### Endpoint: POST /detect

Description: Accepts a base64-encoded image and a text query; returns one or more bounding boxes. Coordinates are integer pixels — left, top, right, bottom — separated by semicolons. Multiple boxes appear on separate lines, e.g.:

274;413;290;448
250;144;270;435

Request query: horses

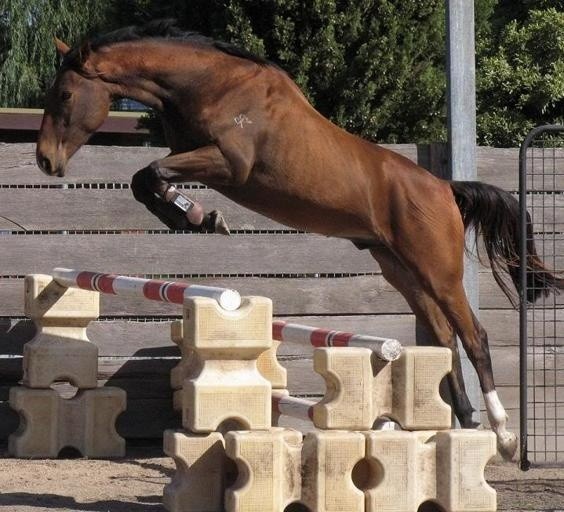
36;11;564;461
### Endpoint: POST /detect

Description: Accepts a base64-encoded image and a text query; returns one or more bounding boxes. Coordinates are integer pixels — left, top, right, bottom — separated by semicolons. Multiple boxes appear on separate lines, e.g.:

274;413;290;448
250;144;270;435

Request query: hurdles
9;267;498;512
